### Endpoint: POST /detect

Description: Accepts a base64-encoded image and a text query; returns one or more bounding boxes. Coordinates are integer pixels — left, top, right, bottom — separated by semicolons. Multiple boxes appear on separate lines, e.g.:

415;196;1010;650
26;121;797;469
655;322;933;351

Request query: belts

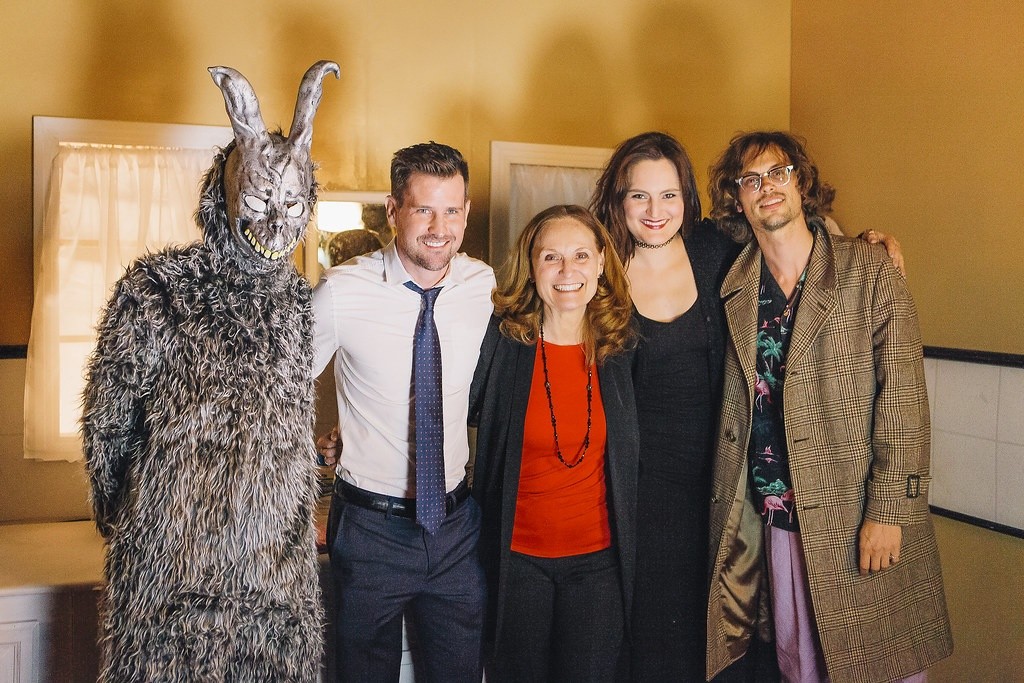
331;476;472;520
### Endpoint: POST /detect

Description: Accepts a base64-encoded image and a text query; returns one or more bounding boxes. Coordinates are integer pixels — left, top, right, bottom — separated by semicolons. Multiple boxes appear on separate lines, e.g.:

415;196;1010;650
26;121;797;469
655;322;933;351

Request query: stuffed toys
81;59;320;683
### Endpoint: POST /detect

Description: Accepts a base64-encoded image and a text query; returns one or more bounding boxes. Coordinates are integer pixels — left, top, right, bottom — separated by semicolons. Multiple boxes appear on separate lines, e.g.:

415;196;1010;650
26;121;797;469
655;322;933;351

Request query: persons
312;131;932;683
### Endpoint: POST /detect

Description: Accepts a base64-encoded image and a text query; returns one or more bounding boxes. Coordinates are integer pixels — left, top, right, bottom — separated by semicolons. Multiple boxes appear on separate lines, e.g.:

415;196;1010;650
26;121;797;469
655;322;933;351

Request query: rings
889;556;900;559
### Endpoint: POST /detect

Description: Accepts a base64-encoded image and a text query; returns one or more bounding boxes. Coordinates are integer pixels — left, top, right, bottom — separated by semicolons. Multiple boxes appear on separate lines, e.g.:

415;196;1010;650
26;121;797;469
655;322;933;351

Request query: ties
402;280;446;536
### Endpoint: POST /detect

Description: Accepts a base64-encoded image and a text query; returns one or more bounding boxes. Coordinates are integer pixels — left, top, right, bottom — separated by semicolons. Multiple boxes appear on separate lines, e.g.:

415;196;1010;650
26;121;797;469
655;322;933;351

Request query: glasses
733;166;795;195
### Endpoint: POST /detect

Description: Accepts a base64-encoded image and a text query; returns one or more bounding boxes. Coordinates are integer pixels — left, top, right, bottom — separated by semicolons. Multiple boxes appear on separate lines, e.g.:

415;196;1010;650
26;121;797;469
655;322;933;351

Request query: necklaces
540;301;622;468
629;231;679;250
